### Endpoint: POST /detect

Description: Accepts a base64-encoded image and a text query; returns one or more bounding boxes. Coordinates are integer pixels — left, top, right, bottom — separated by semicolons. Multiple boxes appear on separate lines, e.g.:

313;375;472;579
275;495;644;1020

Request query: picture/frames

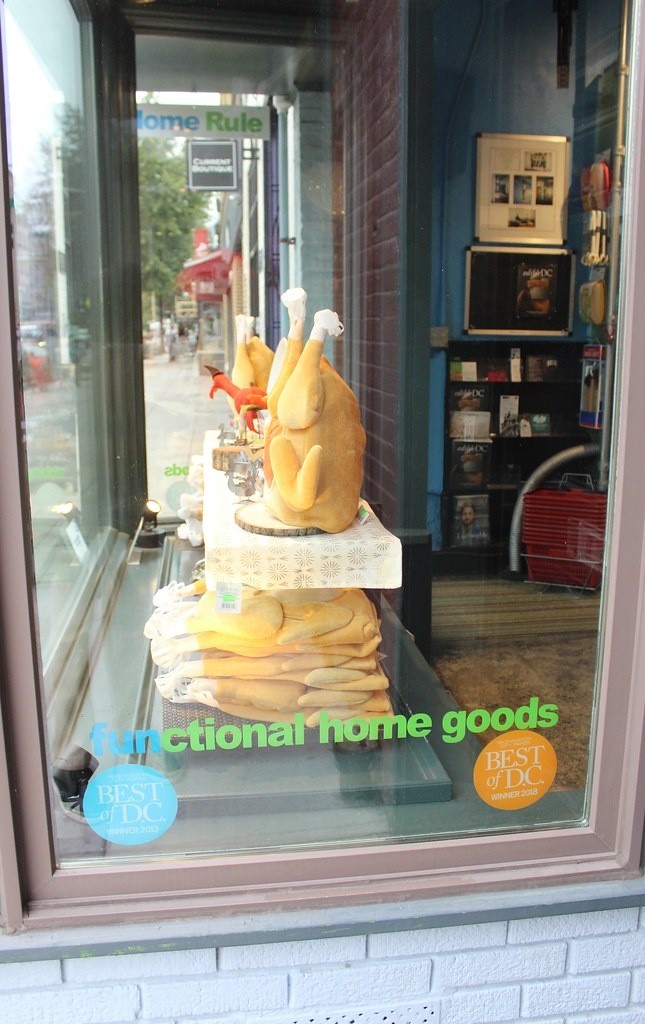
463;244;577;337
473;131;571;245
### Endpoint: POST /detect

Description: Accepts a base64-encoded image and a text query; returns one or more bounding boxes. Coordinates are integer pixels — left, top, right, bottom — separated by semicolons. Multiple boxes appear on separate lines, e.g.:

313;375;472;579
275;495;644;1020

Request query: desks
201;428;403;754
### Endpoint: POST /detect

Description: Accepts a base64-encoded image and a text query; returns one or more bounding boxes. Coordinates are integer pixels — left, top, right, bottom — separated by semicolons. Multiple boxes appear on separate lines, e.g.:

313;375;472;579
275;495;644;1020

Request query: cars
143;334;159;357
19;321;92;381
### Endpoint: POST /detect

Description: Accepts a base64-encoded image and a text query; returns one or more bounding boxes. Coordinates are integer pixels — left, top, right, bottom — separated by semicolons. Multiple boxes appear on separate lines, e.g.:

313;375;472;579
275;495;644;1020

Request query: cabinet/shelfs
439;341;588;548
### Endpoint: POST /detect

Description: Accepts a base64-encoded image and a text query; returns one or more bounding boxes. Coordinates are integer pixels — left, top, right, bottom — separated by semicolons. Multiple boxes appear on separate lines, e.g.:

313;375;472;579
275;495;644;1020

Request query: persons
42;630;125;824
452;502;489;546
164;312;176;360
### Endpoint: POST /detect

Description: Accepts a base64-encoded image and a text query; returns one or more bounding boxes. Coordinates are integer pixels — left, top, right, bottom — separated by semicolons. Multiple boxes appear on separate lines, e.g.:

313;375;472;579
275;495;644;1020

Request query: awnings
177;251;236;303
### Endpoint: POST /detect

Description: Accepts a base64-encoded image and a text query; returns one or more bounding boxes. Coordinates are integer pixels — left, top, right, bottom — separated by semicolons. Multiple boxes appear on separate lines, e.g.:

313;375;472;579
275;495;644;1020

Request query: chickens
147;564;392;736
211;285;367;535
